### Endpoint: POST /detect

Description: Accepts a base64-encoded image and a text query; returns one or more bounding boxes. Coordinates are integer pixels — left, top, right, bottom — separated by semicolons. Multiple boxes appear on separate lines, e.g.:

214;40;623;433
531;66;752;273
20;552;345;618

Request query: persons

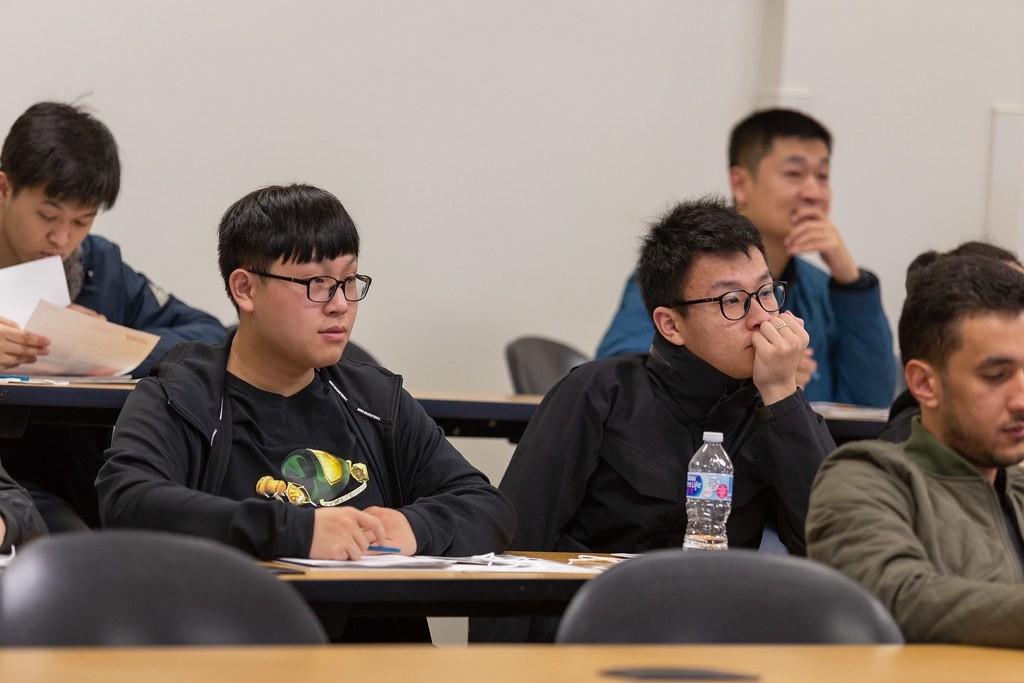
0;102;230;565
467;196;838;645
805;241;1024;649
596;108;898;409
95;182;517;646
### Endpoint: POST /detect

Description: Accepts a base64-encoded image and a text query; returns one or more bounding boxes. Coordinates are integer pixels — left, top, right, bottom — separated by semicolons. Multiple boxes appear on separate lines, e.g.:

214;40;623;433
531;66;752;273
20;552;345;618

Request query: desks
0;645;1024;683
251;549;754;618
0;375;890;444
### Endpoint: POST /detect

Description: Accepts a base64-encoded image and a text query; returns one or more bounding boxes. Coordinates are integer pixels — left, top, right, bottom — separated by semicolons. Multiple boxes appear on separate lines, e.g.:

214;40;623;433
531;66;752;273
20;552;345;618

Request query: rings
777;323;789;329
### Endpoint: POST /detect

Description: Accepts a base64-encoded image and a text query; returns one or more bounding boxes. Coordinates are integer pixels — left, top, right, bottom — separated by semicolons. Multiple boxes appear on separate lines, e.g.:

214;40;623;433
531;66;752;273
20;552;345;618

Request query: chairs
0;530;326;649
505;334;591;396
556;548;903;647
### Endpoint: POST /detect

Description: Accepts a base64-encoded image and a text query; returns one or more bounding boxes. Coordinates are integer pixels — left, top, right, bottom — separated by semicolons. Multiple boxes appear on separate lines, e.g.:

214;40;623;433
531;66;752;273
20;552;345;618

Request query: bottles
683;433;733;551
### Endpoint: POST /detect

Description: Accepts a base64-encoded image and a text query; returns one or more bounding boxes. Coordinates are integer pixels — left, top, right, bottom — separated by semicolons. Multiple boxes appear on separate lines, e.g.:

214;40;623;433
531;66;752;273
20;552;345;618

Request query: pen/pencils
368;546;400;551
813;372;820;380
0;375;30;381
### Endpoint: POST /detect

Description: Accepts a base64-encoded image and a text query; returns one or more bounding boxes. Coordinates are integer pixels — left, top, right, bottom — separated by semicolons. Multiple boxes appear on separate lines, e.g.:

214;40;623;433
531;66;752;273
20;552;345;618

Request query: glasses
246;268;373;303
675;281;789;320
